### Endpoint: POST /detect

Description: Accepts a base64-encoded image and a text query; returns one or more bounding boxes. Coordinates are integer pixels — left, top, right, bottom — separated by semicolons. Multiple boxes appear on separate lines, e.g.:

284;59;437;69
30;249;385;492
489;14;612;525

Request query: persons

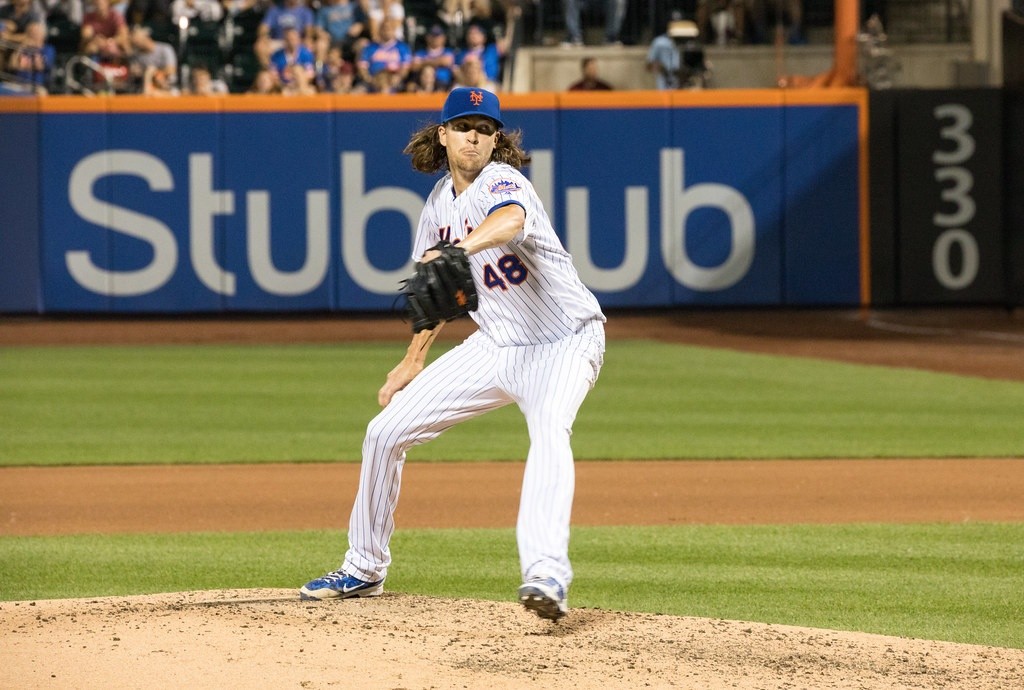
852;13;900;91
566;58;612;91
0;0;546;94
300;86;608;618
645;12;713;89
563;0;808;44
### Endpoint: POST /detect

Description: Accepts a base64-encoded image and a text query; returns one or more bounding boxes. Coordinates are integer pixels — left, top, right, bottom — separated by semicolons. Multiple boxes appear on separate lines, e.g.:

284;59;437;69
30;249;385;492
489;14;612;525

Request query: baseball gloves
392;236;480;333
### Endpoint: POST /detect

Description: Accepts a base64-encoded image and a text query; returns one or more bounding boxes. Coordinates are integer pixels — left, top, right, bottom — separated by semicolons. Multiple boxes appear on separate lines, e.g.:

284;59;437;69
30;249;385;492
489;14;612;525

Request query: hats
441;87;505;128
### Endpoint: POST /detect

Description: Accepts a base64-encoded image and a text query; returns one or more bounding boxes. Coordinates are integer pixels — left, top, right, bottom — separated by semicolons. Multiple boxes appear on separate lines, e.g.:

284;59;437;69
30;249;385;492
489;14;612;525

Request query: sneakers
517;576;568;618
299;568;386;600
